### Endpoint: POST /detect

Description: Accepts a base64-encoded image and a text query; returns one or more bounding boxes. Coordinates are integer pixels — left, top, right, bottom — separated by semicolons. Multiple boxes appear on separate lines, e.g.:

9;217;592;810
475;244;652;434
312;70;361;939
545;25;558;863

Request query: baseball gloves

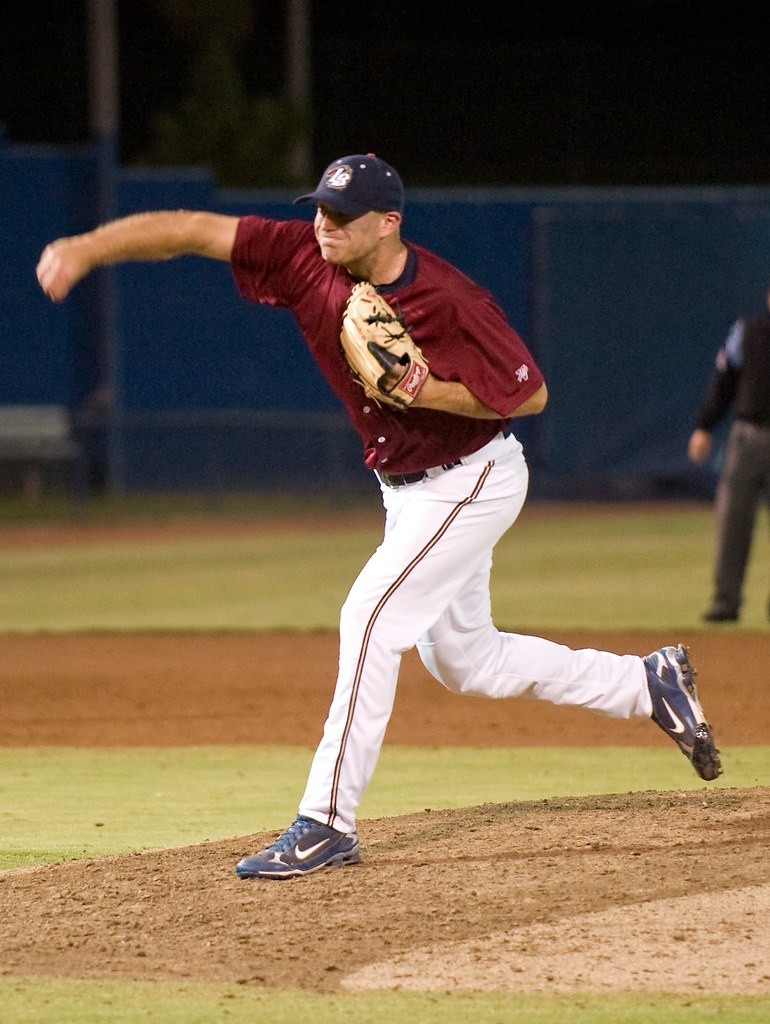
337;282;432;412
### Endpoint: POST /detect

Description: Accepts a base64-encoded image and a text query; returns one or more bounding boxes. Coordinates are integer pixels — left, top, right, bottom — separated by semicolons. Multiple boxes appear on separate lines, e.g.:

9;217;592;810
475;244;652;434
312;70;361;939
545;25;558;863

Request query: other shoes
703;609;737;622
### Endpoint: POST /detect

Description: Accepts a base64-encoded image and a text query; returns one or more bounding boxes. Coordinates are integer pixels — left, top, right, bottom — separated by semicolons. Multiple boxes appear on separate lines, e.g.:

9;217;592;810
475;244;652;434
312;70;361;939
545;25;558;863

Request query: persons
193;0;259;143
35;150;726;881
686;307;769;622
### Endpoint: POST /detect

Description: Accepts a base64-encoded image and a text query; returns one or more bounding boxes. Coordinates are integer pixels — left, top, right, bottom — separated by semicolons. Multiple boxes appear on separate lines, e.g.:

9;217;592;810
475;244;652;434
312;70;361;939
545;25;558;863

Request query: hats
293;153;405;216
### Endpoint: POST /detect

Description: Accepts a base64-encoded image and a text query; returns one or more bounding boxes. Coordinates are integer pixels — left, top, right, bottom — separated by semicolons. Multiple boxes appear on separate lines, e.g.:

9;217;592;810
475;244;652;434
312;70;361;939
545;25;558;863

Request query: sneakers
643;643;723;781
235;817;359;879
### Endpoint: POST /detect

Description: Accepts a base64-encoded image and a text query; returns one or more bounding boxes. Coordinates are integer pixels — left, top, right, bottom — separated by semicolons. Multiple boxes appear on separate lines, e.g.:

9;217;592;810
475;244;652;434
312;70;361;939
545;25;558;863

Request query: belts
376;426;512;489
